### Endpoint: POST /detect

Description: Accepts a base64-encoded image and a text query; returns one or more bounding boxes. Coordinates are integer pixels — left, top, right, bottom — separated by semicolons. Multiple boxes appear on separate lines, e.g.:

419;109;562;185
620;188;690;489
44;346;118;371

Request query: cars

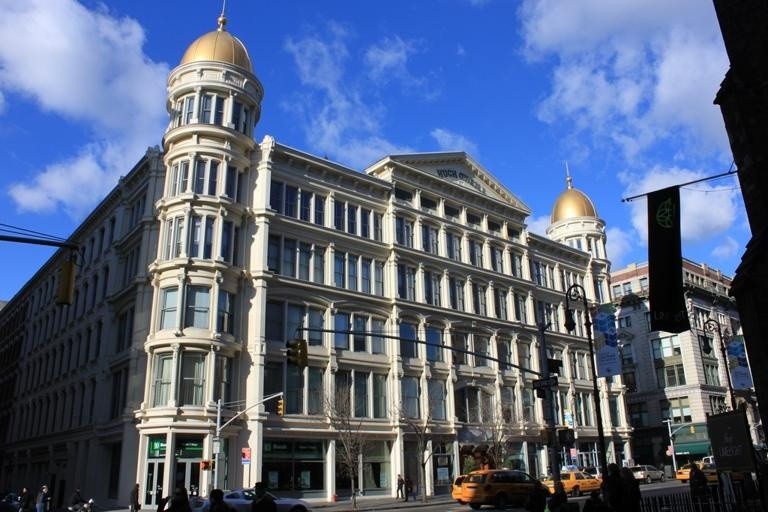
191;489;312;512
676;456;718;483
452;465;666;510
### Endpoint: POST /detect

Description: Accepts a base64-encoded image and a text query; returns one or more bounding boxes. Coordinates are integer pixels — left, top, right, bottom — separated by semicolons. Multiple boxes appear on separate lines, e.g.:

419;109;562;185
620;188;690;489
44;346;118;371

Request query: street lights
564;283;609;481
703;318;736;410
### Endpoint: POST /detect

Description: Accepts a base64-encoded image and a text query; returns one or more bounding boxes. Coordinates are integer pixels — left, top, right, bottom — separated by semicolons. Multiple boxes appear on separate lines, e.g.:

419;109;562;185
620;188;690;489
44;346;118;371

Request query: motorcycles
63;499;95;512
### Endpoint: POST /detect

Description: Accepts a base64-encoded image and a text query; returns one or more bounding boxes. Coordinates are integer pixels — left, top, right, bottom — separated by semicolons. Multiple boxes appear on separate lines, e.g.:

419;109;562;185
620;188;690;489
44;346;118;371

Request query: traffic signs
533;377;558;389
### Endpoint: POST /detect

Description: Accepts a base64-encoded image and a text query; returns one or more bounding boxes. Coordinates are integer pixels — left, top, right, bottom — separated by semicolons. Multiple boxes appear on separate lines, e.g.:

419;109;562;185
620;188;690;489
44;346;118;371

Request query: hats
255;483;268;488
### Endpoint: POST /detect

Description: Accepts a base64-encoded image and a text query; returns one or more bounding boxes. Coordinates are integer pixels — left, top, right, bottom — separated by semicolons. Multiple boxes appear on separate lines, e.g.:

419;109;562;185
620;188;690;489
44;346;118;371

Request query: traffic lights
275;399;286;417
286;339;308;367
691;426;696;434
200;461;211;470
55;263;76;304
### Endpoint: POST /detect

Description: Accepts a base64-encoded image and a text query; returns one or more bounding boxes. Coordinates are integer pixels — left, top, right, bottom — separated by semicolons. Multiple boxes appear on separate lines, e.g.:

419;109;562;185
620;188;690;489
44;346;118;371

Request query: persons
156;486;192;512
17;487;30;512
401;477;417;502
396;474;404;500
547;462;642;512
207;489;230;512
251;481;277;512
689;464;711;512
35;485;52;512
128;484;139;512
71;487;88;512
